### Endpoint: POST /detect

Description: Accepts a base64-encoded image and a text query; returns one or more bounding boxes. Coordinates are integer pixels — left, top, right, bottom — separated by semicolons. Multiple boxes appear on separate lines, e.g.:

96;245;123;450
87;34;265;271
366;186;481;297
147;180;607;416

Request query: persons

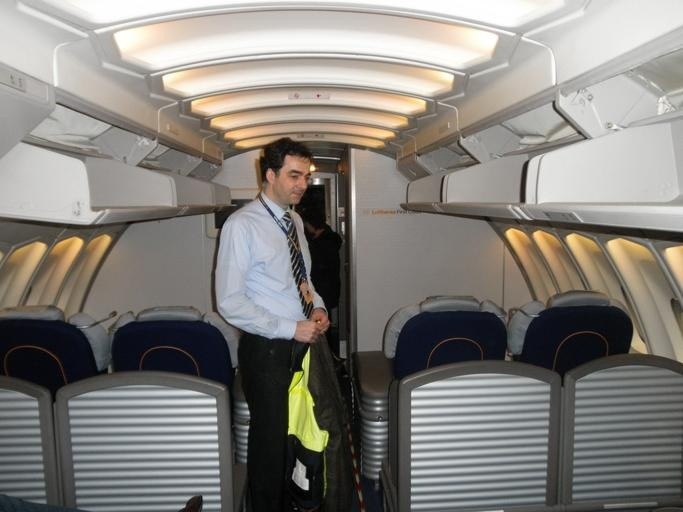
214;137;330;512
304;215;342;349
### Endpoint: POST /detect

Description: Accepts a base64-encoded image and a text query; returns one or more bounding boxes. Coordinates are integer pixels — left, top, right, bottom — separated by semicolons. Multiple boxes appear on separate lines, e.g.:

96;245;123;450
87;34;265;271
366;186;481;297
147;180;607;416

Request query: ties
281;212;314;320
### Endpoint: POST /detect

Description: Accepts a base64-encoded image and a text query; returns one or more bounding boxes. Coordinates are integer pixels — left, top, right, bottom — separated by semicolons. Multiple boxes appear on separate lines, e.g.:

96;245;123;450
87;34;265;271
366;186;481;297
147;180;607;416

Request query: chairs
3;376;63;508
506;289;635;388
380;360;561;511
351;294;508;512
555;354;680;511
4;304;112;405
106;304;251;473
52;371;252;511
